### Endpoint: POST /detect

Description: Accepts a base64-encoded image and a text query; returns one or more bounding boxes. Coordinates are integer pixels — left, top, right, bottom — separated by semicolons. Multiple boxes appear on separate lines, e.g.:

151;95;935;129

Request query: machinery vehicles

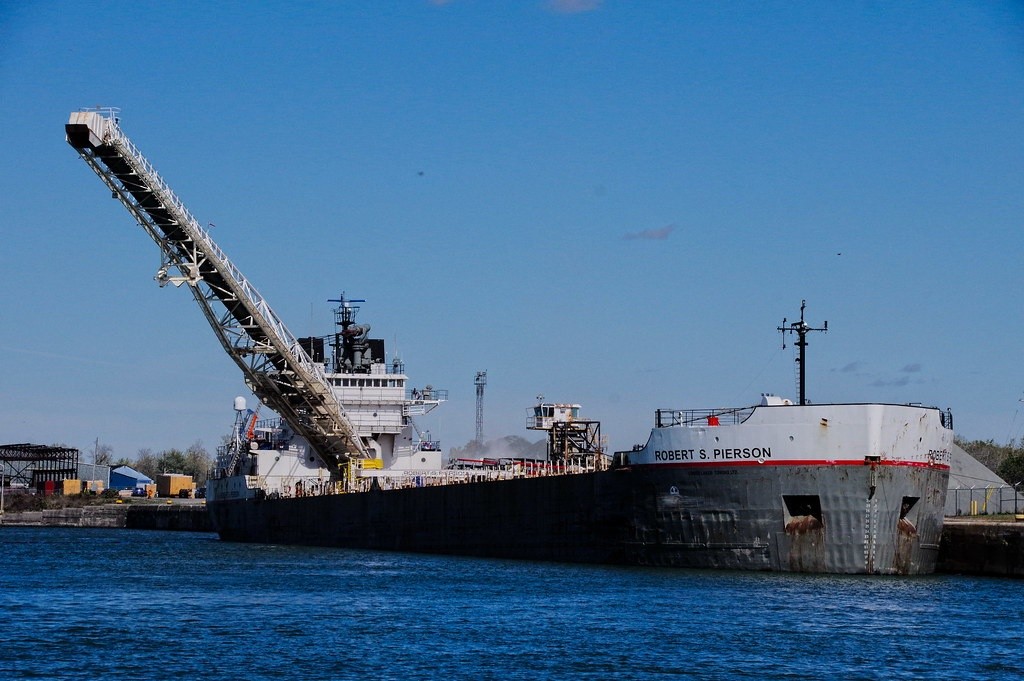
65;105;384;482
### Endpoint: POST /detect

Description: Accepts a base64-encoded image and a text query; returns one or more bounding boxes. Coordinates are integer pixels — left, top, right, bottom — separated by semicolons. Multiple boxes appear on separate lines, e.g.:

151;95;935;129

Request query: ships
204;292;956;578
